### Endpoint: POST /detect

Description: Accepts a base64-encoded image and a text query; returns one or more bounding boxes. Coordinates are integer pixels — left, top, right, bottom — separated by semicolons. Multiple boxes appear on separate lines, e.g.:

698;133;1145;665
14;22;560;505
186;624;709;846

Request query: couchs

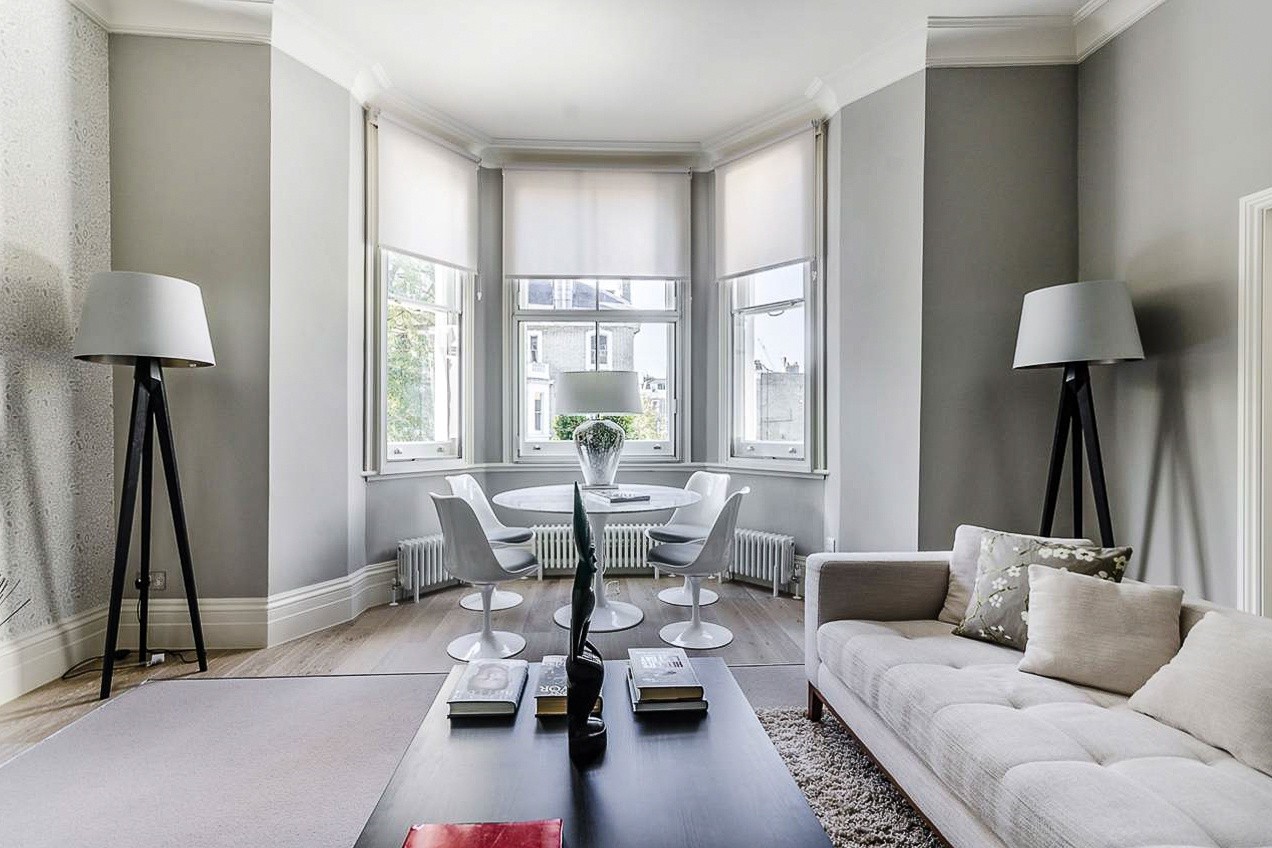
805;552;1272;848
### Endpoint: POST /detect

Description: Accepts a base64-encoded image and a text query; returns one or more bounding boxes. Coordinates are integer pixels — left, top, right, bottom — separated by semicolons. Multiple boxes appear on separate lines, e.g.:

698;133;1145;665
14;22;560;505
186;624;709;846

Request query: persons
467;659;528;695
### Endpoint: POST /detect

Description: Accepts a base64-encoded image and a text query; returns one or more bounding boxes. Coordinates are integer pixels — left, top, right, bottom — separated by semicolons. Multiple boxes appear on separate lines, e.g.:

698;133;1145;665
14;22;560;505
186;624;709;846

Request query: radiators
390;524;802;606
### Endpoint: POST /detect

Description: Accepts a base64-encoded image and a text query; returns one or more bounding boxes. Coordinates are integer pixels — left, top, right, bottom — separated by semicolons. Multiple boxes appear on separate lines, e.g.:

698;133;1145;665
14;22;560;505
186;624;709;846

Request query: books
588;489;650;503
626;648;708;713
446;659;529;717
401;818;564;848
534;655;603;717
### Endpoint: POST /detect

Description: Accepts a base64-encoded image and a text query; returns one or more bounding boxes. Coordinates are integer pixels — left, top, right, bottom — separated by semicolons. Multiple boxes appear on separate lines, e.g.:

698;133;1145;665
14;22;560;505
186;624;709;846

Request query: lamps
554;371;643;487
1012;281;1144;548
74;271;217;700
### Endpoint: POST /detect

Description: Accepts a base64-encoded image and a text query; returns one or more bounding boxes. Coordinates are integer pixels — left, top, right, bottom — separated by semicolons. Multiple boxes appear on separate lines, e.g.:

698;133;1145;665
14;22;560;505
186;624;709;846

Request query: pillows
1125;610;1272;778
1017;564;1185;696
938;525;1093;625
951;530;1133;652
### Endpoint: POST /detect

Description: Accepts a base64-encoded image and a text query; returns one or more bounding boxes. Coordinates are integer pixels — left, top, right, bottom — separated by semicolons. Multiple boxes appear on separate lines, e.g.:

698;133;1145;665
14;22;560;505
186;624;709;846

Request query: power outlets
827;537;834;553
137;571;166;590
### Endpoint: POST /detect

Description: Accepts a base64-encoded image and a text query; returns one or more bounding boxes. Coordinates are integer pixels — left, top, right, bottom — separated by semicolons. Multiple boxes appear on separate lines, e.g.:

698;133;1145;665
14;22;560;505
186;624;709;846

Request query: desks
492;483;702;631
355;657;832;848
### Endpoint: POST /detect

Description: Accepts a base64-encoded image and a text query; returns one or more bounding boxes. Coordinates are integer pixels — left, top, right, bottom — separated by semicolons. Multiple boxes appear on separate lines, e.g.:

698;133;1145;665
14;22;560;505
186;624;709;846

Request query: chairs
646;487;751;649
446;474;536;610
429;493;540;661
646;470;731;606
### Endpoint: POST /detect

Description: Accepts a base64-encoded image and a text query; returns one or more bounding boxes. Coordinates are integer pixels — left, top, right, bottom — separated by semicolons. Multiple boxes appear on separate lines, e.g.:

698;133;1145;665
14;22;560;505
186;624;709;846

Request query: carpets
753;706;949;848
0;662;807;848
